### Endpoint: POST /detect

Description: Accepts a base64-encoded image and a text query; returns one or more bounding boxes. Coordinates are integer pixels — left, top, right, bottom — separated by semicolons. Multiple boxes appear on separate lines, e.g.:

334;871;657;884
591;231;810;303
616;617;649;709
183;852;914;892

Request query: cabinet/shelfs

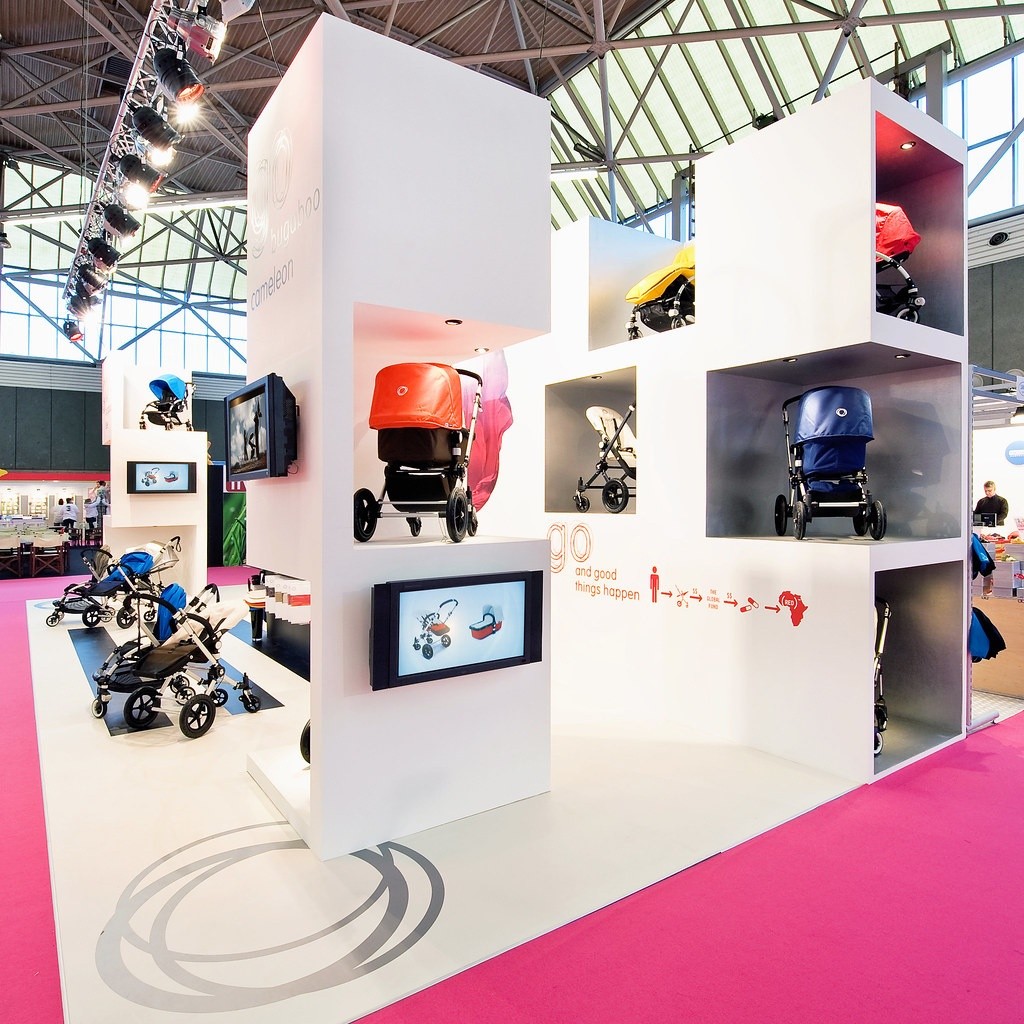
973;594;1024;699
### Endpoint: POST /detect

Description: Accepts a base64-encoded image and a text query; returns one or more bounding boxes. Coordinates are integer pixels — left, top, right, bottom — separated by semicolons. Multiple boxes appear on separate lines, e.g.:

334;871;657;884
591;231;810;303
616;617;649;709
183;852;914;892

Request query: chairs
0;534;66;580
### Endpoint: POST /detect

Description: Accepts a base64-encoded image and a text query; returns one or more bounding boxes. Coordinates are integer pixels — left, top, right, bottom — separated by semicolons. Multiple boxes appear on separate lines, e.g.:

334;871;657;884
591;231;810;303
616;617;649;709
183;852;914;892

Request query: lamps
59;1;254;340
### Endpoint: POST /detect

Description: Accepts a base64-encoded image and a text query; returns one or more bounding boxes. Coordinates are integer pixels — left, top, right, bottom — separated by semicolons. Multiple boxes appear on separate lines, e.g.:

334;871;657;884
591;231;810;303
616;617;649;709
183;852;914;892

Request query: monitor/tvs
981;513;1004;533
369;570;542;691
126;461;196;495
224;373;298;482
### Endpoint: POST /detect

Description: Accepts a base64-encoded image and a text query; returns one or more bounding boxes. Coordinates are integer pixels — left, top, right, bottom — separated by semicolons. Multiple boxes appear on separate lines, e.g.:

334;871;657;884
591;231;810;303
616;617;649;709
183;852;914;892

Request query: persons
89;481;100;496
973;481;1008;526
102;499;111;515
83;496;101;529
52;499;65;527
96;482;105;529
59;498;79;528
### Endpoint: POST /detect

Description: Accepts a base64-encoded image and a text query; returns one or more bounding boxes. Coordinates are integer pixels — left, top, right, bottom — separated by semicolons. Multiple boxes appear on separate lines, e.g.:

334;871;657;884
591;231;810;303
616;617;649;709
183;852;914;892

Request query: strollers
627;240;698;337
572;398;637;514
772;384;891;540
45;536;226;663
140;373;196;432
876;201;926;323
92;595;263;737
142;467;161;487
874;599;893;757
412;599;459;660
353;360;485;544
